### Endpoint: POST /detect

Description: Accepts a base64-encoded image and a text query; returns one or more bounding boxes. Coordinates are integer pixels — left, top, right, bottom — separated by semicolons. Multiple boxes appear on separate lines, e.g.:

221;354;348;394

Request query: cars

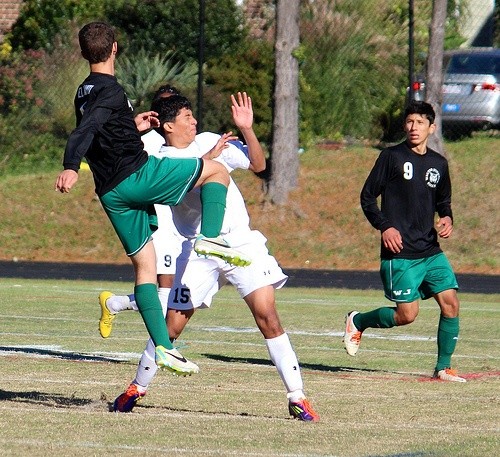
406;47;500;137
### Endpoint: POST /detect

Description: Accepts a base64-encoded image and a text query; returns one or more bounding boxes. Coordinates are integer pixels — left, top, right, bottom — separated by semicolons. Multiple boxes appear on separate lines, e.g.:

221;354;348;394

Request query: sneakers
154;346;200;377
112;385;147;412
98;291;116;337
194;234;253;266
432;368;466;382
342;310;361;356
288;399;321;422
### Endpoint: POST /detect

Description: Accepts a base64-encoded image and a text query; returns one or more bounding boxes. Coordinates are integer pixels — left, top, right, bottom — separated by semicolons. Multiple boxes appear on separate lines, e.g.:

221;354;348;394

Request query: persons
342;101;467;382
56;20;252;377
99;84;182;339
113;91;321;422
